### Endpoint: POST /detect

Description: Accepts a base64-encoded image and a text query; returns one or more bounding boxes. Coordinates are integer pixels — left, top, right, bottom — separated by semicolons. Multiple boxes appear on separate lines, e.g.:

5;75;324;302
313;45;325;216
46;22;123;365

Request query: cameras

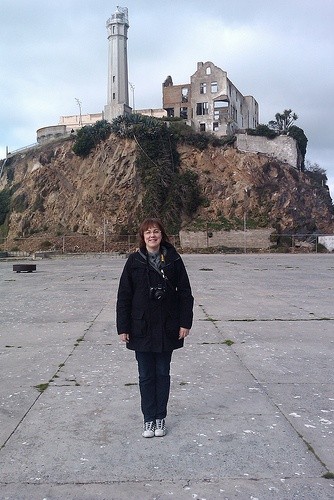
150;287;167;301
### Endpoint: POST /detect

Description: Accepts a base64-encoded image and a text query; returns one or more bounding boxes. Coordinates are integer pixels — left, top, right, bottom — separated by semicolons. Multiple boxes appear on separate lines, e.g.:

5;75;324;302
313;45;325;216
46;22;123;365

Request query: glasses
143;230;162;235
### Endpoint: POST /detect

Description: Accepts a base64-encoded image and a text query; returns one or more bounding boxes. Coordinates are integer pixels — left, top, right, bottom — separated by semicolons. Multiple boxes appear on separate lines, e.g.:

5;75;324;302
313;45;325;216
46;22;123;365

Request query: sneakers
142;420;155;438
155;419;166;436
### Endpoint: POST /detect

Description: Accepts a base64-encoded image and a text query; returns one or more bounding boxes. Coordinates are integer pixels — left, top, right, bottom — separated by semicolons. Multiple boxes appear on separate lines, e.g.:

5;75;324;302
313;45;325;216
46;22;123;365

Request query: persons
115;218;195;439
